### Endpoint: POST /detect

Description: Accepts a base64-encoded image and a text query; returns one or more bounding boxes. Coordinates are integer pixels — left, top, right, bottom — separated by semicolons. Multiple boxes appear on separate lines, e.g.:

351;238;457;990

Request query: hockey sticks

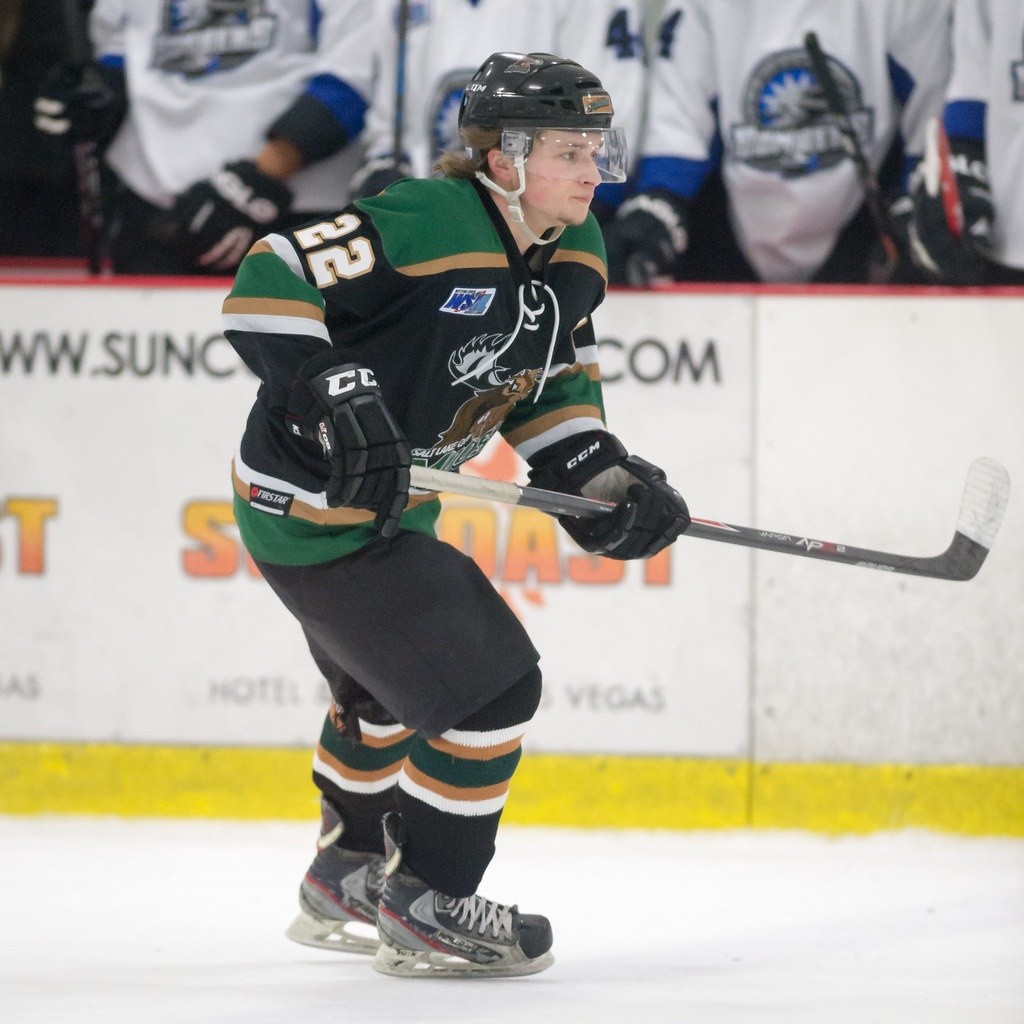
49;0;109;275
409;452;1012;583
803;30;967;281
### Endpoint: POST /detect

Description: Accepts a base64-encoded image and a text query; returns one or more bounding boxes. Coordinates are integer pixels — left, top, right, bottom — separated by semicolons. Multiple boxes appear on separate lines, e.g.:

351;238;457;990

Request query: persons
223;47;693;980
0;0;1024;287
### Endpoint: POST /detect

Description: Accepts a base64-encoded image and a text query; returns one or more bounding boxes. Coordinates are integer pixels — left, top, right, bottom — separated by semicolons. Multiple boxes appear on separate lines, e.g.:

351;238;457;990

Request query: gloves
33;61;120;143
527;431;692;561
610;193;689;286
172;161;287;275
282;347;412;538
892;143;993;283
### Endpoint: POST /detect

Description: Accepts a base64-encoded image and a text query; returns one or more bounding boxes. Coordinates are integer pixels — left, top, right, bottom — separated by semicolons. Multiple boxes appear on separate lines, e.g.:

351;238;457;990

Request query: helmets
457;51;615;158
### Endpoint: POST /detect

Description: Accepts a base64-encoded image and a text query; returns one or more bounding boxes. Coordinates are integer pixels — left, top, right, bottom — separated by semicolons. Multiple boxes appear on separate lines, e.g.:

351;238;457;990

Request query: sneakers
374;810;554;976
287;796;390;954
